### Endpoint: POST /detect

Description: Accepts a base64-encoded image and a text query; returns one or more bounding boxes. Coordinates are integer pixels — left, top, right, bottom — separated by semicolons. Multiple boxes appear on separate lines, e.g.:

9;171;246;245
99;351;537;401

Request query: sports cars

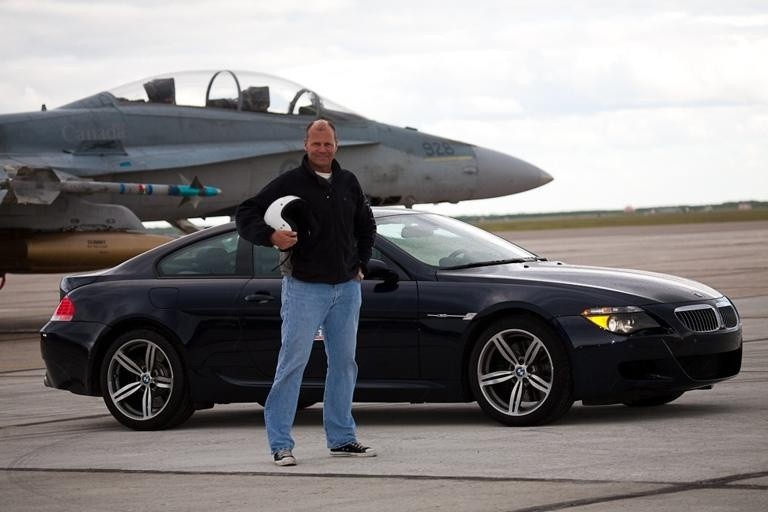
36;209;743;432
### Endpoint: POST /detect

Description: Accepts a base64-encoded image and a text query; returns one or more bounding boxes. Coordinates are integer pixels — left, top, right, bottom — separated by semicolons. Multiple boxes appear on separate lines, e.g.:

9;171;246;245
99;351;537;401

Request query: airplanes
2;65;552;298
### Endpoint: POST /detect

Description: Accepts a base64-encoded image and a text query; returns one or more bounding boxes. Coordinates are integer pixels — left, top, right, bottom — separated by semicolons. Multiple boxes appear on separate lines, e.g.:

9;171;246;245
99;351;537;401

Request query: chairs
196;246;235;275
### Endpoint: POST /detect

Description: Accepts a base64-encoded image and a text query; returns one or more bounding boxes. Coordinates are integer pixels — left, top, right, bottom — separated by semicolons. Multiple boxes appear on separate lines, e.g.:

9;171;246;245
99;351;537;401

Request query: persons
236;117;377;466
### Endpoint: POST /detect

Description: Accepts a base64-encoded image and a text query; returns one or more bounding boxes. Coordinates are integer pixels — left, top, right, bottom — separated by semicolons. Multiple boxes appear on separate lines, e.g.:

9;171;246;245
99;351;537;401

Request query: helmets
264;195;321;252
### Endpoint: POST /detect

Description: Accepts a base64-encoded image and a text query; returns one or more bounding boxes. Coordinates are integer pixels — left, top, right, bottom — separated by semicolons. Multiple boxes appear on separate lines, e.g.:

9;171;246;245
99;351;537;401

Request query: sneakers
273;449;299;467
330;441;378;458
